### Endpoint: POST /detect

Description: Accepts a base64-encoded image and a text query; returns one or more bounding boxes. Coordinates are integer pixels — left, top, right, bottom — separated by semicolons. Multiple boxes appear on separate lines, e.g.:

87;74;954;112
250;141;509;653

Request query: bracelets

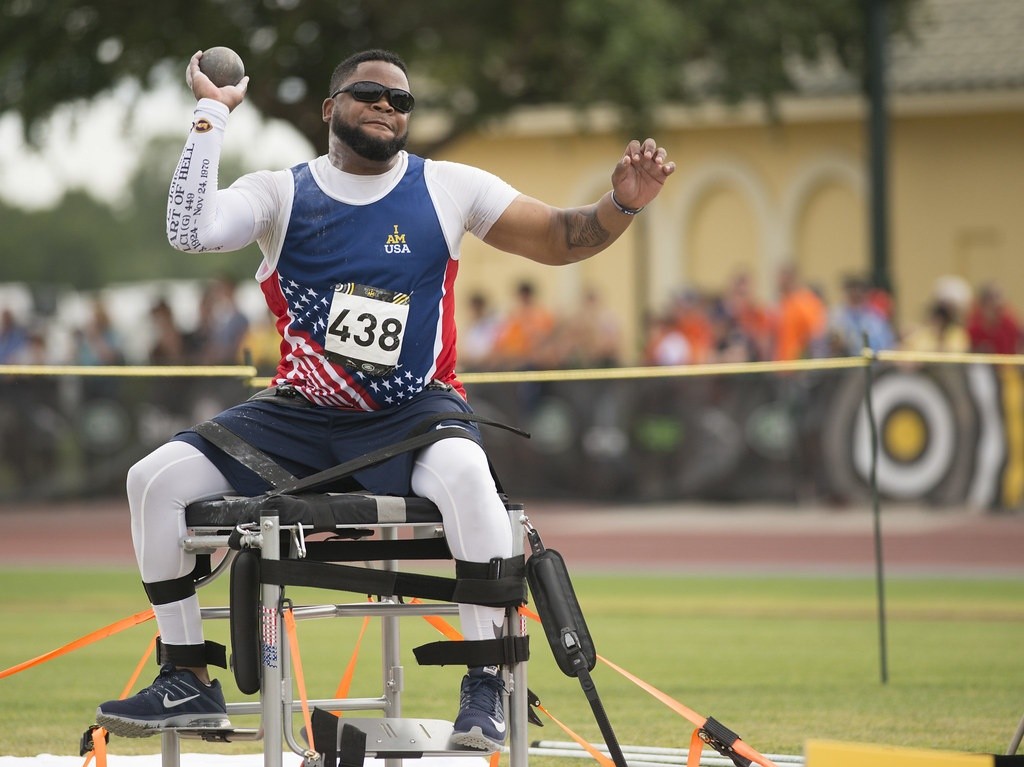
610;188;644;215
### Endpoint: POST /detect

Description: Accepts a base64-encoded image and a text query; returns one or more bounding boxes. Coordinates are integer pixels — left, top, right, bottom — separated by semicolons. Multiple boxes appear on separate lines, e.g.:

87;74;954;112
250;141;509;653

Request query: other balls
198;45;245;89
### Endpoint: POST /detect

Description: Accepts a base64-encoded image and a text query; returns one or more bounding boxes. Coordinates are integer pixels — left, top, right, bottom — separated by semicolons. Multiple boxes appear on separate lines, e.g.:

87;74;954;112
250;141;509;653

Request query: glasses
330;80;416;114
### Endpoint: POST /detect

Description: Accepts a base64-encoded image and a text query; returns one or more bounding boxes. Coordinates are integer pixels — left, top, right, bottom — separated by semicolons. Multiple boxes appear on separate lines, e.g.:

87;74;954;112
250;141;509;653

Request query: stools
160;489;532;767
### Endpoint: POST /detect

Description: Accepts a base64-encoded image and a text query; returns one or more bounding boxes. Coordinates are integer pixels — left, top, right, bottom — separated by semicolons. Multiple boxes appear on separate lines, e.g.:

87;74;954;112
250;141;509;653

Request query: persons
0;293;253;364
452;267;1024;373
94;48;675;753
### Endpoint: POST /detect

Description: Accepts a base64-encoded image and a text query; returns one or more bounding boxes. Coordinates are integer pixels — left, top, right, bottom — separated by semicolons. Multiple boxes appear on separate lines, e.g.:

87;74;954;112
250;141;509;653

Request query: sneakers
450;665;509;752
96;665;231;739
